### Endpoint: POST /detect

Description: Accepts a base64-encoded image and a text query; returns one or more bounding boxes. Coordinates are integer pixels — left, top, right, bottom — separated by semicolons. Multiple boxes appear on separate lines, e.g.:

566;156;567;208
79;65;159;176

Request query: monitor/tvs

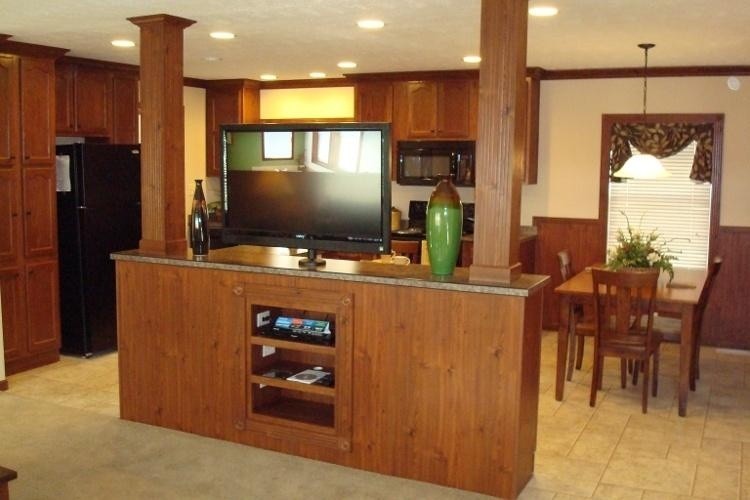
219;121;392;266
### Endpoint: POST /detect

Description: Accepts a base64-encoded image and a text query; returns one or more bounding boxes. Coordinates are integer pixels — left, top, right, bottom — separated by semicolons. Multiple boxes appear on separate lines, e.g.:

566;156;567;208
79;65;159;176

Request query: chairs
555;249;725;416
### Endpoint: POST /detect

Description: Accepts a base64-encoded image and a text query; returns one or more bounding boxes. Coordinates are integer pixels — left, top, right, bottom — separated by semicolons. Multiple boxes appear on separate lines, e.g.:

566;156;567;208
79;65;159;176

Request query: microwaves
396;141;476;187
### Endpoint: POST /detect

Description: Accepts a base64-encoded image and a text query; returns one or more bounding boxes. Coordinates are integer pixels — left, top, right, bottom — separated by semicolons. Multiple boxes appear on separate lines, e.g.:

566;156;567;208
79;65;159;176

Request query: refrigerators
54;140;141;359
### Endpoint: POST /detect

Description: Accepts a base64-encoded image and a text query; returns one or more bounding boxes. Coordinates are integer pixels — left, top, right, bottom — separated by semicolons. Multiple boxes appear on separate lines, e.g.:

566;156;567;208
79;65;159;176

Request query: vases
425;175;464;277
190;178;210;256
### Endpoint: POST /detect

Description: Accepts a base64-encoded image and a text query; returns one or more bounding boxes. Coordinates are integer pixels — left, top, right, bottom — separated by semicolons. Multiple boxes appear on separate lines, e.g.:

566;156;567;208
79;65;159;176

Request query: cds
296;374;317;380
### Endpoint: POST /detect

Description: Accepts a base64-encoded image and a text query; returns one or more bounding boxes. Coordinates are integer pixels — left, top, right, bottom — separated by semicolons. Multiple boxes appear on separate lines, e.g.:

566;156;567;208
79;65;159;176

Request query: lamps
612;42;674;180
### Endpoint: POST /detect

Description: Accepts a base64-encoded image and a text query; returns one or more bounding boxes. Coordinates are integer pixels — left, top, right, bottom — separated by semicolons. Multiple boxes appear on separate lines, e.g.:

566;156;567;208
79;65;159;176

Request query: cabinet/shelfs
353;82;392;123
0;34;70;379
56;59;112;137
250;335;334;434
394;80;476;141
85;76;137;145
204;87;259;176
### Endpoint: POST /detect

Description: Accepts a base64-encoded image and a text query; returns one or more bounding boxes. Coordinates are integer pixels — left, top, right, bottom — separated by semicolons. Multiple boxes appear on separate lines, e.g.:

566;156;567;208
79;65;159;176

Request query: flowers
601;210;692;288
